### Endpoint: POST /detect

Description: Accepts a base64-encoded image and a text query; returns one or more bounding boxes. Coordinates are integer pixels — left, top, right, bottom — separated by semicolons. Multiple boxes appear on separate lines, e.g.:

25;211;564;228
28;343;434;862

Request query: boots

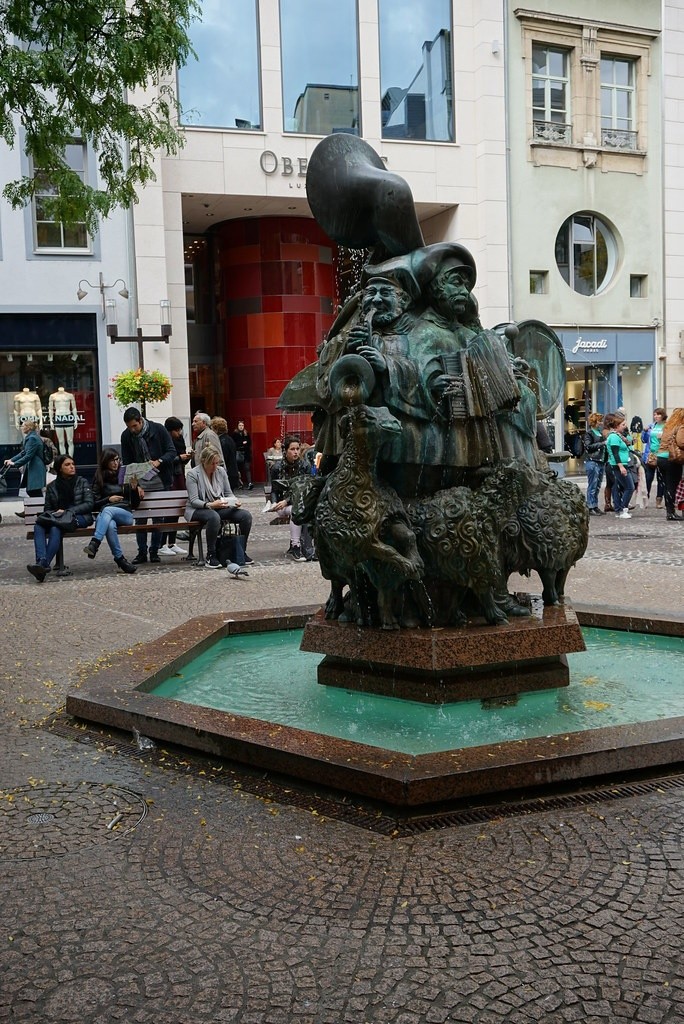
83;538;101;559
114;555;137;574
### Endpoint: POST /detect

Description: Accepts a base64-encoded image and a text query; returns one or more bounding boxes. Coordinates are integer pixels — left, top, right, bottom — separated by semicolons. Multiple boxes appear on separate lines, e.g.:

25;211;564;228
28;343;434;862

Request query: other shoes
589;495;684;521
27;564;51;583
248;483;255;490
239;485;244;490
15;511;25;518
150;552;161;563
132;554;147;565
176;533;190;542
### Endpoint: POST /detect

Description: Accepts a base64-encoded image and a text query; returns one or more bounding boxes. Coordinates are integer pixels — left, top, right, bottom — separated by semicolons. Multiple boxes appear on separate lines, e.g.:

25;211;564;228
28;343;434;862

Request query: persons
176;410;228;540
120;406;176;564
263;435;322;561
232;420;255;490
27;454;93;582
308;237;537;497
3;419;58;518
184;442;254;569
83;448;141;573
13;387;43;443
49;387;78;458
583;405;684;522
211;417;239;493
564;405;585;458
156;417;197;555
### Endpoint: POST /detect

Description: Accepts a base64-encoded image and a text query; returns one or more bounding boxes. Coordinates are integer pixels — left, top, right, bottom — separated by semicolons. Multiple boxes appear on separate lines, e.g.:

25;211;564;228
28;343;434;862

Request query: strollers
0;464;11;522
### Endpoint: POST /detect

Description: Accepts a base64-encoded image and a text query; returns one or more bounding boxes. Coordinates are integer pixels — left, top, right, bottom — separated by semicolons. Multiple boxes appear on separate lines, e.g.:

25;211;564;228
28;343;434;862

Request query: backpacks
34;434;58;465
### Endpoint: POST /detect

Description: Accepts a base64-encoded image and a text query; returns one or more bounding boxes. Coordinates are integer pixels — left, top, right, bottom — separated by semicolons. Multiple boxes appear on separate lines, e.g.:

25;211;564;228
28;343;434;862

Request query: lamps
77;272;130;321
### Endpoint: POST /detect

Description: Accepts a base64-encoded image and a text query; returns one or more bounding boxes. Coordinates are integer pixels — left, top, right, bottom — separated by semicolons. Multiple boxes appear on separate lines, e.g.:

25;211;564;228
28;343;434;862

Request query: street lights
106;324;173;422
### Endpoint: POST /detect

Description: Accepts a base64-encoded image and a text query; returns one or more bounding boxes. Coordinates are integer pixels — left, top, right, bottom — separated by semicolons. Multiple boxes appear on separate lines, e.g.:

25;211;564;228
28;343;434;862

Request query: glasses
108;458;122;465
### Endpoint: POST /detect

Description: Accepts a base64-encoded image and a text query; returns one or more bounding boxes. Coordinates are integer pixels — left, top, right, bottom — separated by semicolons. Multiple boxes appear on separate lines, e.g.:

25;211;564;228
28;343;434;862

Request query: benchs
264;485;311;559
21;488;206;569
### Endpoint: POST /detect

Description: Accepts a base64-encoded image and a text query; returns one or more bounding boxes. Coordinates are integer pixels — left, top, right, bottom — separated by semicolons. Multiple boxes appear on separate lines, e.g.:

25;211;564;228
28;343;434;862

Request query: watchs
159;458;162;463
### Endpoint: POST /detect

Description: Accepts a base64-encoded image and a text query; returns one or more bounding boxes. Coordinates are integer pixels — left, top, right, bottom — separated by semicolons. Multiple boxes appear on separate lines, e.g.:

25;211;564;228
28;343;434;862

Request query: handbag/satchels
36;509;79;533
236;451;245;461
645;453;658;465
627;451;635;468
215;520;245;565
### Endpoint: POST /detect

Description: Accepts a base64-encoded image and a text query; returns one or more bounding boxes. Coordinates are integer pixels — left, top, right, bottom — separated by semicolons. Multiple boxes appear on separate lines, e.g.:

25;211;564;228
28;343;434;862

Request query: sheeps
277;403;588;630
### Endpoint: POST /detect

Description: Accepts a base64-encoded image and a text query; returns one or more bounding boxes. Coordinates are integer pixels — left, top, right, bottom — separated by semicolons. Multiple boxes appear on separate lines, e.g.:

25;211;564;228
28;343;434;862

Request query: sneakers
157;545;176;556
205;555;222;569
244;552;254;564
287;545;307;562
170;544;188;555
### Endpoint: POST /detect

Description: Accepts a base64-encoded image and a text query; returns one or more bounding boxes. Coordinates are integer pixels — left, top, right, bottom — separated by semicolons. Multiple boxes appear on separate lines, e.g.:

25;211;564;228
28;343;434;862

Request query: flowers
107;368;173;407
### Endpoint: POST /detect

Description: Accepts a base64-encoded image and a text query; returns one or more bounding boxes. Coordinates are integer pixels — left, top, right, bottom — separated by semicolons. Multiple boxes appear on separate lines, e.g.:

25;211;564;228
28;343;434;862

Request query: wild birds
226;559;249;580
